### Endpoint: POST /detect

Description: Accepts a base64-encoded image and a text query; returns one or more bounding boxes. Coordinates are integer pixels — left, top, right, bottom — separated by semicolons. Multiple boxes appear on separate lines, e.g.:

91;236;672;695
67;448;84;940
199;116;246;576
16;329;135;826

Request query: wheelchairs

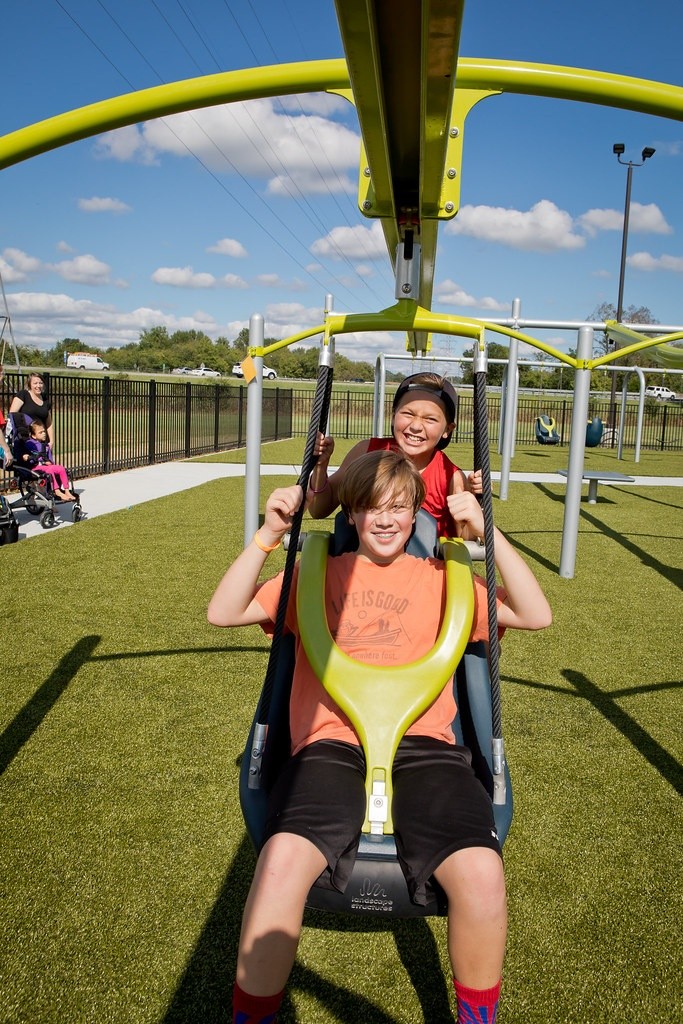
5;413;82;528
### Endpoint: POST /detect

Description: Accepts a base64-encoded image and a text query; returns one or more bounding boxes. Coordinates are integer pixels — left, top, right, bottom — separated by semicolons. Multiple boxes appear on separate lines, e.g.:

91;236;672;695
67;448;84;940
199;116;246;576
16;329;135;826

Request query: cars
192;368;222;377
173;366;193;375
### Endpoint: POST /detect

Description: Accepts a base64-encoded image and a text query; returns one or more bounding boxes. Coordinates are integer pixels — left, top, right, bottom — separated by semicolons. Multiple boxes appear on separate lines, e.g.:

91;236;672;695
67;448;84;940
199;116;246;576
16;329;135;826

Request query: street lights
604;143;656;429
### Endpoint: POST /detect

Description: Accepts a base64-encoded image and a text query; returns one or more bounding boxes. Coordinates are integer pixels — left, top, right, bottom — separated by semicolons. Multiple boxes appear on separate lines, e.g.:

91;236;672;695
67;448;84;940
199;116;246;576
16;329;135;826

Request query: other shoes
58;492;76;502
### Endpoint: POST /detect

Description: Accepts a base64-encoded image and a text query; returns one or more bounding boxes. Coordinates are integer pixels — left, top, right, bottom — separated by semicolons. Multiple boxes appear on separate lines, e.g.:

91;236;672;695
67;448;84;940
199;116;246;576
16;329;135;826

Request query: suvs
645;386;677;399
354;378;365;382
232;361;277;380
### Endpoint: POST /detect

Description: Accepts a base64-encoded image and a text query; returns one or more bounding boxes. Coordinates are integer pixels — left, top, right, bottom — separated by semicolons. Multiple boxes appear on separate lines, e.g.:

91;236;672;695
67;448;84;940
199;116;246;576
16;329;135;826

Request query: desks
557;470;635;505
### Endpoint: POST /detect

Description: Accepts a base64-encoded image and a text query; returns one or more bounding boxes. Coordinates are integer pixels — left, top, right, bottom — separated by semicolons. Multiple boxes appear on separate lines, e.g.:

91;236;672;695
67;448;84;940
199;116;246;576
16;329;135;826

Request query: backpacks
0;428;15;471
0;495;20;546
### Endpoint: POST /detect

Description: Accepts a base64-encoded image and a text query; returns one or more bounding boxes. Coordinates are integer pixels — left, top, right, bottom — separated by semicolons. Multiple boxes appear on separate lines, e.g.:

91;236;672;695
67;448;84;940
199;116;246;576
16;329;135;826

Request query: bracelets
254;530;280;554
308;474;329;493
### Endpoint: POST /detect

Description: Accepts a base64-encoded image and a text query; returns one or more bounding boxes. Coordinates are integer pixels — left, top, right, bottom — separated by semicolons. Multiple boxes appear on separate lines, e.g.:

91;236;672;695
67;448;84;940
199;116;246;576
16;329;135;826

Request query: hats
393;372;458;451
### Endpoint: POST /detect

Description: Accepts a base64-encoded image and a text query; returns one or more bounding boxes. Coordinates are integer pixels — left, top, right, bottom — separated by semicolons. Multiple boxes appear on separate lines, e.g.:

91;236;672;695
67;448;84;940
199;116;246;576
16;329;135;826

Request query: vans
67;352;110;370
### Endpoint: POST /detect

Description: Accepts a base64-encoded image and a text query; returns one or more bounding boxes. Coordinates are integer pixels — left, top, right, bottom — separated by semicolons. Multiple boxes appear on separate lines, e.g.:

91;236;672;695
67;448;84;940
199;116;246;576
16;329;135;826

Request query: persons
307;372;493;541
207;450;552;1024
9;372;52;443
0;364;14;467
20;420;76;501
